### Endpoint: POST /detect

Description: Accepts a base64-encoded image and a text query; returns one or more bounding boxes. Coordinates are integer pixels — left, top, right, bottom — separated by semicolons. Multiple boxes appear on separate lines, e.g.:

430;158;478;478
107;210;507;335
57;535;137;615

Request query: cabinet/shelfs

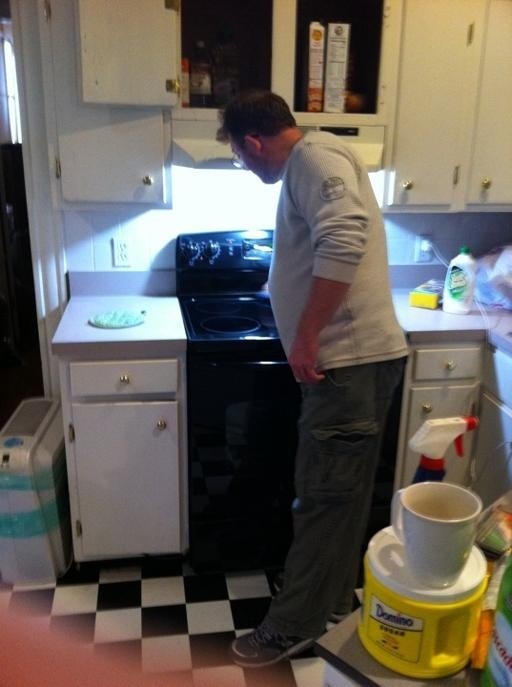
391;339;512;514
382;0;511;215
39;1;177;206
178;1;398;169
53;353;189;564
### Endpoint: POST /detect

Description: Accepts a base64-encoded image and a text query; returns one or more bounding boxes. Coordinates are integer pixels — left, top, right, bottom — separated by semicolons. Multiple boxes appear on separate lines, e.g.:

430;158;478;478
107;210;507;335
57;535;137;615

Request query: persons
313;30;321;41
216;86;409;668
331;26;346;42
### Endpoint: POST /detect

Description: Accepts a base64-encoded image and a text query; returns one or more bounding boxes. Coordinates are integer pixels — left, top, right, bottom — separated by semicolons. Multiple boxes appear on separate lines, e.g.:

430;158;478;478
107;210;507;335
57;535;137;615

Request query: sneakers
271;570;352;623
228;623;327;669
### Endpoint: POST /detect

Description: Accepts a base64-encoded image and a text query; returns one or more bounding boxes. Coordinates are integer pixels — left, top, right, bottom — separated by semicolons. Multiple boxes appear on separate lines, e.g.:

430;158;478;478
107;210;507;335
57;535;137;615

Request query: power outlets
414;233;435;263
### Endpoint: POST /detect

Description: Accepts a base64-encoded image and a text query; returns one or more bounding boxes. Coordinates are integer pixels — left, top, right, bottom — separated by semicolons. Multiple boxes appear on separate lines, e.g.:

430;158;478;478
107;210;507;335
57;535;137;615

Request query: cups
391;480;485;589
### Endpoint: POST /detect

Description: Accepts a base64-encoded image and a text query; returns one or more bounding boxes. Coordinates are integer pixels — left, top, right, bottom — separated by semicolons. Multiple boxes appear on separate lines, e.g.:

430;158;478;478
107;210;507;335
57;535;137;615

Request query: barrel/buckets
358;524;490;679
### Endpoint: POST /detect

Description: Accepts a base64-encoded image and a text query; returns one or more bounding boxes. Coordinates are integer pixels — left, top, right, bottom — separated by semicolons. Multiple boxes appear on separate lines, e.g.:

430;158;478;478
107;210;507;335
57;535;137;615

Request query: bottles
439;244;478;314
189;39;214;108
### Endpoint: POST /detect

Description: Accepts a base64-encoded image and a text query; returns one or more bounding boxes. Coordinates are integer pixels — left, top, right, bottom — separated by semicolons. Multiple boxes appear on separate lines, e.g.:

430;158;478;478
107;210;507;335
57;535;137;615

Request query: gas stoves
189;283;279;343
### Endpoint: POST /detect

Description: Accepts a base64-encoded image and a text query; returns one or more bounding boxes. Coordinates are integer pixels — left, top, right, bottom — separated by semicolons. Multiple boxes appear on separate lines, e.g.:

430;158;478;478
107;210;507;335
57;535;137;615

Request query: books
325;22;351;112
305;19;325;110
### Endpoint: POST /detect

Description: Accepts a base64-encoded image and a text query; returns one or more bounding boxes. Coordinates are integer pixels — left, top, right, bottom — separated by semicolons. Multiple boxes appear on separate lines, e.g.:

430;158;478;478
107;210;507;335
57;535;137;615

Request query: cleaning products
408;416;479;485
442;245;476;315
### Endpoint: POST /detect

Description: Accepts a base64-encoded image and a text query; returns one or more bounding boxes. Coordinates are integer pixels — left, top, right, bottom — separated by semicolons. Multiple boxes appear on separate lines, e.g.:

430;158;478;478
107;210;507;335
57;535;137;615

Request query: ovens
186;354;407;577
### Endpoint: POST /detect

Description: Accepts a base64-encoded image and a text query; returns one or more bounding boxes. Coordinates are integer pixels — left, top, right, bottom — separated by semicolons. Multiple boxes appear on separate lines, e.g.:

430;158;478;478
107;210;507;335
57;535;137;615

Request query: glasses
230;134;260;170
